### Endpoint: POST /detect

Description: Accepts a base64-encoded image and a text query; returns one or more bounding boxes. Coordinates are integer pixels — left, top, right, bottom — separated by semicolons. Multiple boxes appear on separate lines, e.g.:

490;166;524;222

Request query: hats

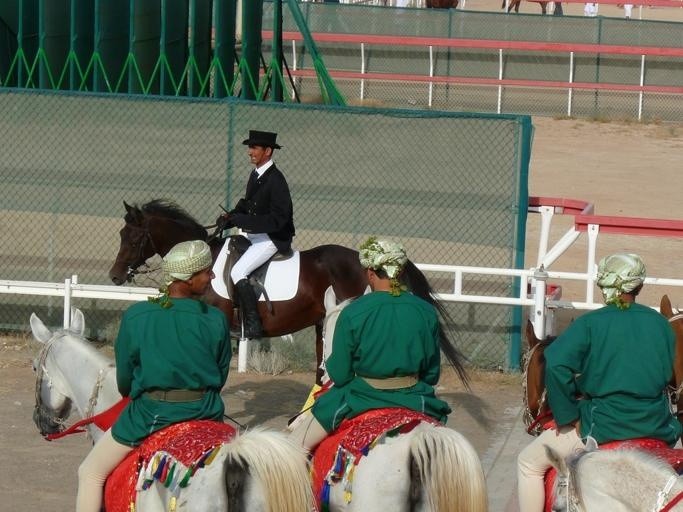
160;239;213;282
594;254;646;294
357;233;407;272
242;130;282;151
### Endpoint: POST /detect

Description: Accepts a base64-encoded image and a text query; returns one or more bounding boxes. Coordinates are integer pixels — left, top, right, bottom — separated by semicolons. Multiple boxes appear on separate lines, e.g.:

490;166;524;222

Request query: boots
231;279;264;339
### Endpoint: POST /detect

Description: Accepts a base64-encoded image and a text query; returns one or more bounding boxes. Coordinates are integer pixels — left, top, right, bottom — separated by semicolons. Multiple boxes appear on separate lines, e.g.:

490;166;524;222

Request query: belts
361;373;419;391
141;389;207;403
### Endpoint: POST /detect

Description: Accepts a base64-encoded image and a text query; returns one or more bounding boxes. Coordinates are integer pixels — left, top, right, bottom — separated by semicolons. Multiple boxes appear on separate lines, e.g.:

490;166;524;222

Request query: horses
281;284;489;512
30;308;319;512
108;197;475;395
521;294;683;512
501;0;549;13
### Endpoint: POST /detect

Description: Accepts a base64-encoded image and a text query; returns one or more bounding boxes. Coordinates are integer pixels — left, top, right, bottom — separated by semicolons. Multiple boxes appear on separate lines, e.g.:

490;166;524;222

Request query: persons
514;252;683;512
282;230;444;465
69;236;234;511
207;129;295;342
